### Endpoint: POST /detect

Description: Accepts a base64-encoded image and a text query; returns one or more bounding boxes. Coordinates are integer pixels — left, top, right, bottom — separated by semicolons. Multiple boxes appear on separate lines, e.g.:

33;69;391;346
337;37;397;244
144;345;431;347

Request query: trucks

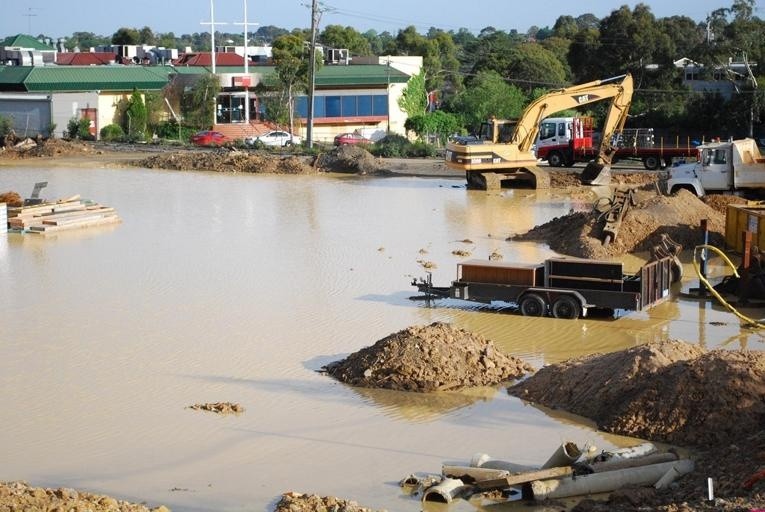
534;112;704;170
662;135;765;199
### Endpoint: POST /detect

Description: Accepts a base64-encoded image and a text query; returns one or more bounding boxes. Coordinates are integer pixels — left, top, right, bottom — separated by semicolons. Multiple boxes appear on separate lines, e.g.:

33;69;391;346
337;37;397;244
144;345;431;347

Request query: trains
641;56;759;99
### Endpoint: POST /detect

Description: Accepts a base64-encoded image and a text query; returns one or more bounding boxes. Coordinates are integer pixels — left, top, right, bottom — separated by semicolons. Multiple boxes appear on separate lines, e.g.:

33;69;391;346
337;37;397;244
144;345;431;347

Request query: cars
190;131;236;149
334;131;378;148
245;130;303;149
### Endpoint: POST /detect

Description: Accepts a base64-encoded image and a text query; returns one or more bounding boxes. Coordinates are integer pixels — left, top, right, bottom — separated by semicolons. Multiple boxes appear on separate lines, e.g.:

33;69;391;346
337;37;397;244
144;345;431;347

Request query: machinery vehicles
440;69;634;190
